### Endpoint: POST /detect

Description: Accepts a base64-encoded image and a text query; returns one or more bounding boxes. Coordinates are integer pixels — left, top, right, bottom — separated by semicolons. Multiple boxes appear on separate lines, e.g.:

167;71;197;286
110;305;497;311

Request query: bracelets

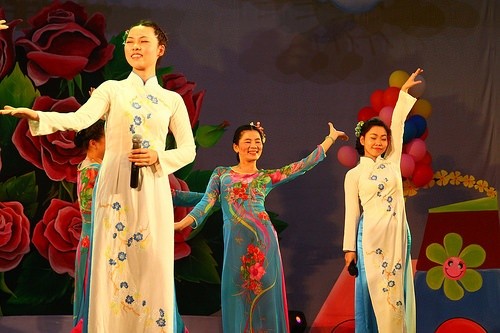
325;134;337;144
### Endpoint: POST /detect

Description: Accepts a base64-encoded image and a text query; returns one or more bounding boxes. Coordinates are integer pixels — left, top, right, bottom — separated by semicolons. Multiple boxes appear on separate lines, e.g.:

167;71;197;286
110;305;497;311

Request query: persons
71;118;217;333
0;19;197;333
342;68;424;333
173;121;348;333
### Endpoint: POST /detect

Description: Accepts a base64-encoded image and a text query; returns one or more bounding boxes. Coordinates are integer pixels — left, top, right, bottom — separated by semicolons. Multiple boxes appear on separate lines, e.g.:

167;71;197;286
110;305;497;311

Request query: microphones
347;261;358;277
130;134;143;189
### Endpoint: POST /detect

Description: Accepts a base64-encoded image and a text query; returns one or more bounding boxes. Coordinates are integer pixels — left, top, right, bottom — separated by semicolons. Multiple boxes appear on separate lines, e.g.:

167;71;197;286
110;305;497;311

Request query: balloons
339;71;434;186
